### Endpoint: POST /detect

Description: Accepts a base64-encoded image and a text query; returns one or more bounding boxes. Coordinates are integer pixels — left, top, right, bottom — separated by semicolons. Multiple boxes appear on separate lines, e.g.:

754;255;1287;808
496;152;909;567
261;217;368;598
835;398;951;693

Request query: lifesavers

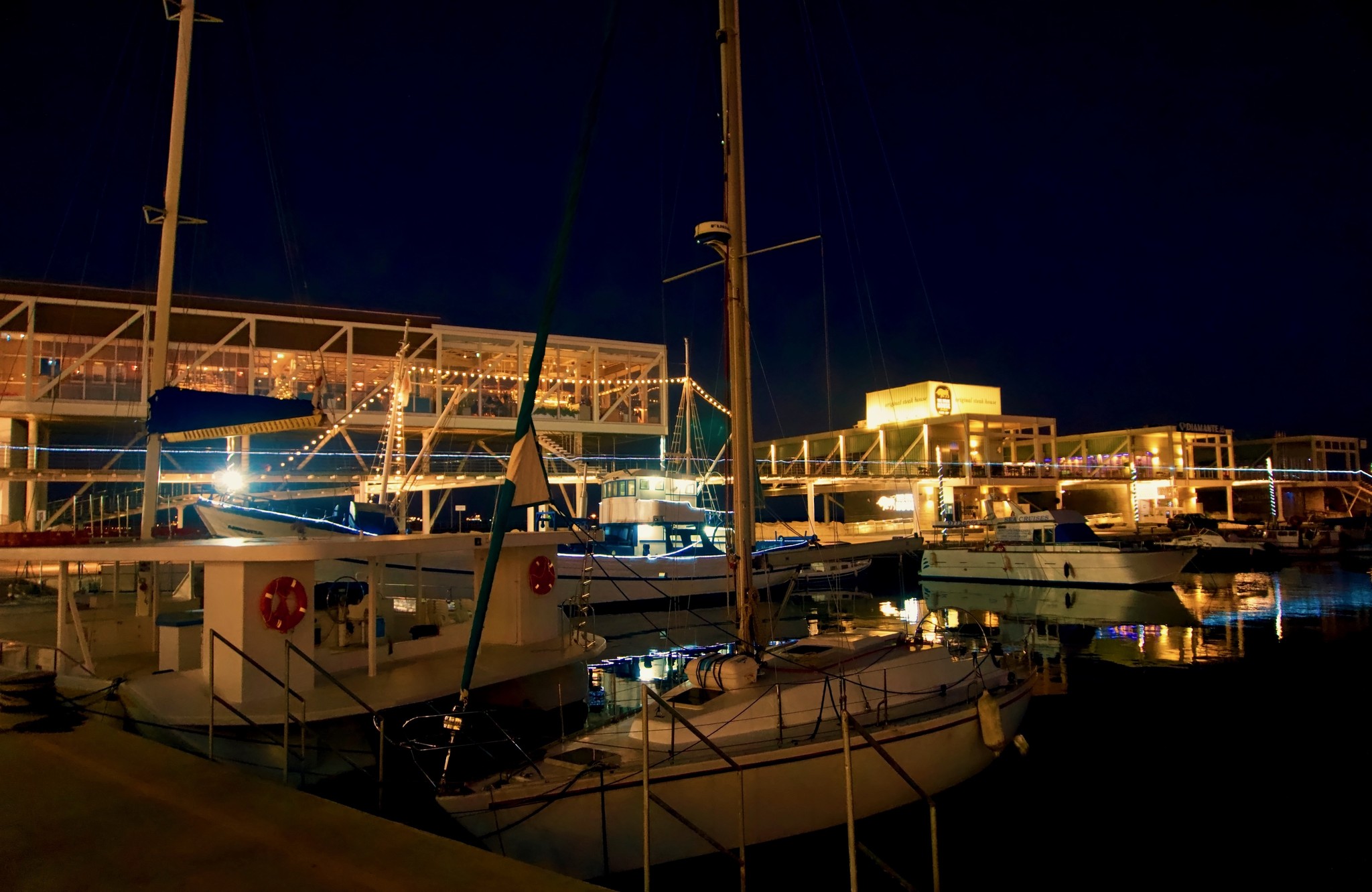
259;574;309;633
992;544;1006;552
528;555;556;596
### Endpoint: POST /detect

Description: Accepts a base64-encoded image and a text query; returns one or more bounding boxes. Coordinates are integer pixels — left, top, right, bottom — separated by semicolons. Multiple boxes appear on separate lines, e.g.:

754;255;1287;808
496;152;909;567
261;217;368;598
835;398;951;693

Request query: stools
156;608;203;676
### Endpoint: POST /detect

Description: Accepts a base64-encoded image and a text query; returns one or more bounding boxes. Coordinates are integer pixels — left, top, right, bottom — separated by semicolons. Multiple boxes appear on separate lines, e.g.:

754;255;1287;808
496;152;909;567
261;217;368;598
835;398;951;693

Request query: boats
1149;511;1279;553
916;491;1199;584
916;577;1203;664
0;528;607;729
427;623;1045;880
